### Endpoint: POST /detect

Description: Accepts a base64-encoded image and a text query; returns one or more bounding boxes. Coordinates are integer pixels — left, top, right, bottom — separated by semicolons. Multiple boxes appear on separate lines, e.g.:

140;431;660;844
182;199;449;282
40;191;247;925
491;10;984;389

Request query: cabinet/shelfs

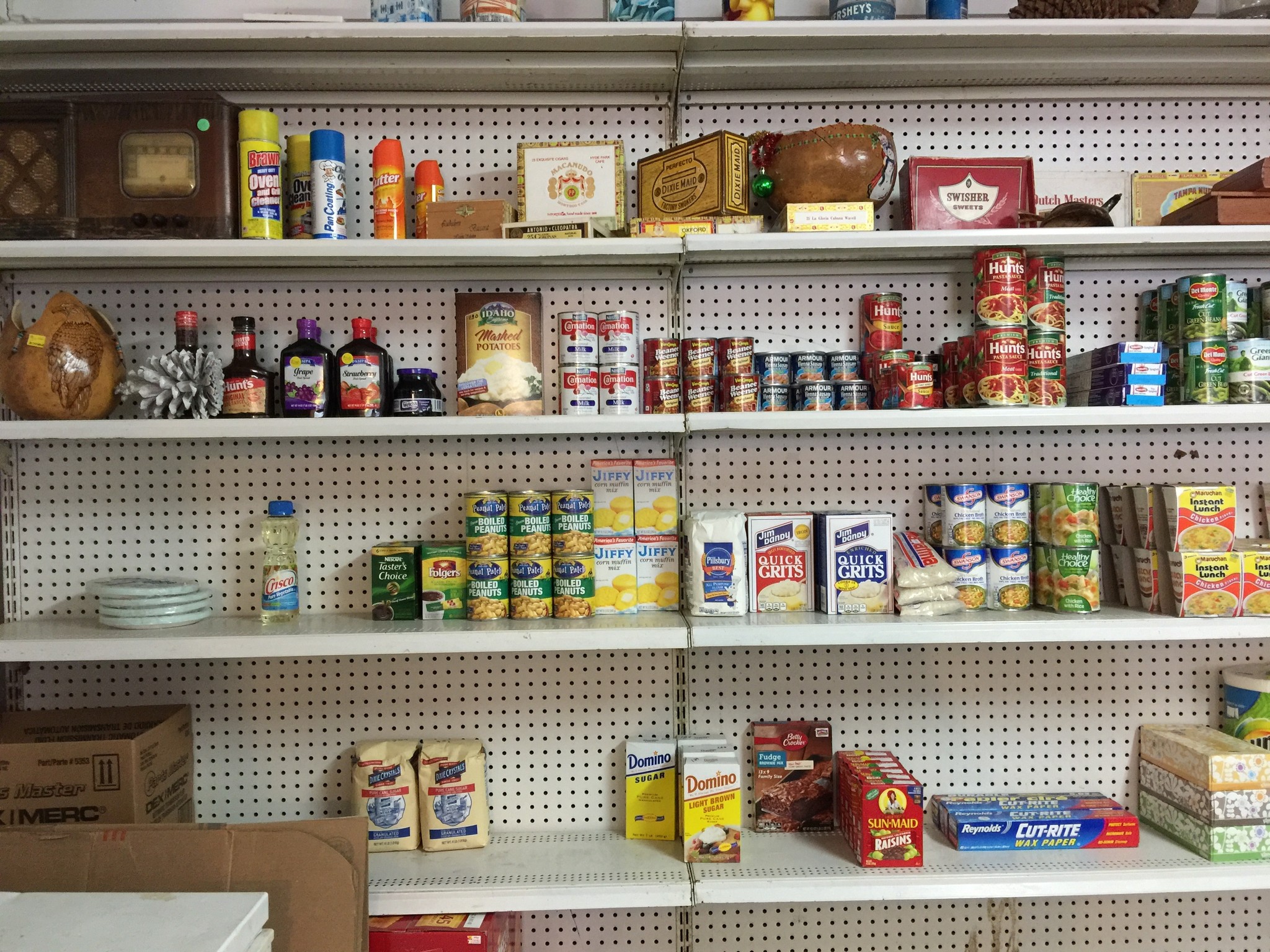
1;2;1268;952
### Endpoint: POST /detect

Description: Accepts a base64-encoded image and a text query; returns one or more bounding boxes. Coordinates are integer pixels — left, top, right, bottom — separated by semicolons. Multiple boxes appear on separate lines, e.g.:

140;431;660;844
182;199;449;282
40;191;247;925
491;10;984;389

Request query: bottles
259;500;302;626
171;307;392;418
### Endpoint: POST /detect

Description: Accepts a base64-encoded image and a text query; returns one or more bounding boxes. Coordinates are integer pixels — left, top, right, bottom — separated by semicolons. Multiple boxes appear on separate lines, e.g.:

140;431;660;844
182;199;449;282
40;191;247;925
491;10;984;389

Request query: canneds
557;245;1269;416
923;481;1101;614
465;489;594;619
